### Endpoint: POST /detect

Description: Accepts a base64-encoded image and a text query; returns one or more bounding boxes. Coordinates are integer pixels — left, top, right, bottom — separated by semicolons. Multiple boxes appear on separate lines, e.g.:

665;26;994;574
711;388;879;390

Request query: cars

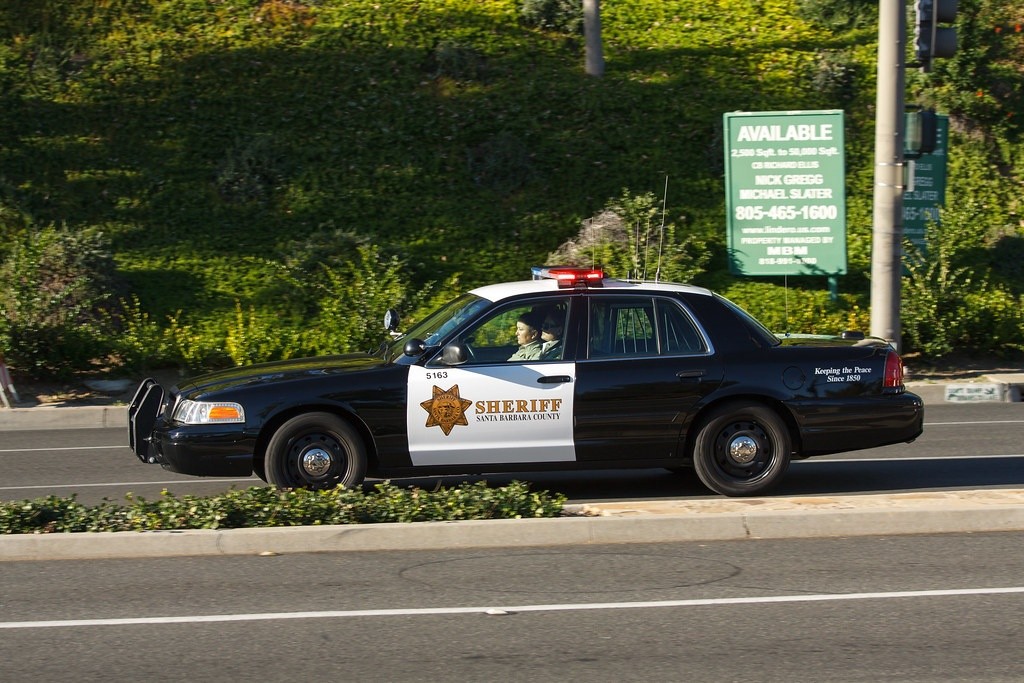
127;266;925;495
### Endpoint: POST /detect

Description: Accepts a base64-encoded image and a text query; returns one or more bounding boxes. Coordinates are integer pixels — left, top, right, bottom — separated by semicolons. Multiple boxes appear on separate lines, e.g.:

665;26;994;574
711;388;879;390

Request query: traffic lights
932;1;960;59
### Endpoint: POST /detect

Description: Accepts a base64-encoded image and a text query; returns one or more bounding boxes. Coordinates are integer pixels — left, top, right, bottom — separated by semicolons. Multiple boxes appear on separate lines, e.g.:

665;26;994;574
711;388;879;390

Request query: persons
507;306;565;360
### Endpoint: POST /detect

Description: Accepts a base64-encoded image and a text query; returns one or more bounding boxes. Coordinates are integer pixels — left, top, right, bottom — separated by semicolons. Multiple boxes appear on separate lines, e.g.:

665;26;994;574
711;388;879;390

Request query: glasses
541;323;558;330
